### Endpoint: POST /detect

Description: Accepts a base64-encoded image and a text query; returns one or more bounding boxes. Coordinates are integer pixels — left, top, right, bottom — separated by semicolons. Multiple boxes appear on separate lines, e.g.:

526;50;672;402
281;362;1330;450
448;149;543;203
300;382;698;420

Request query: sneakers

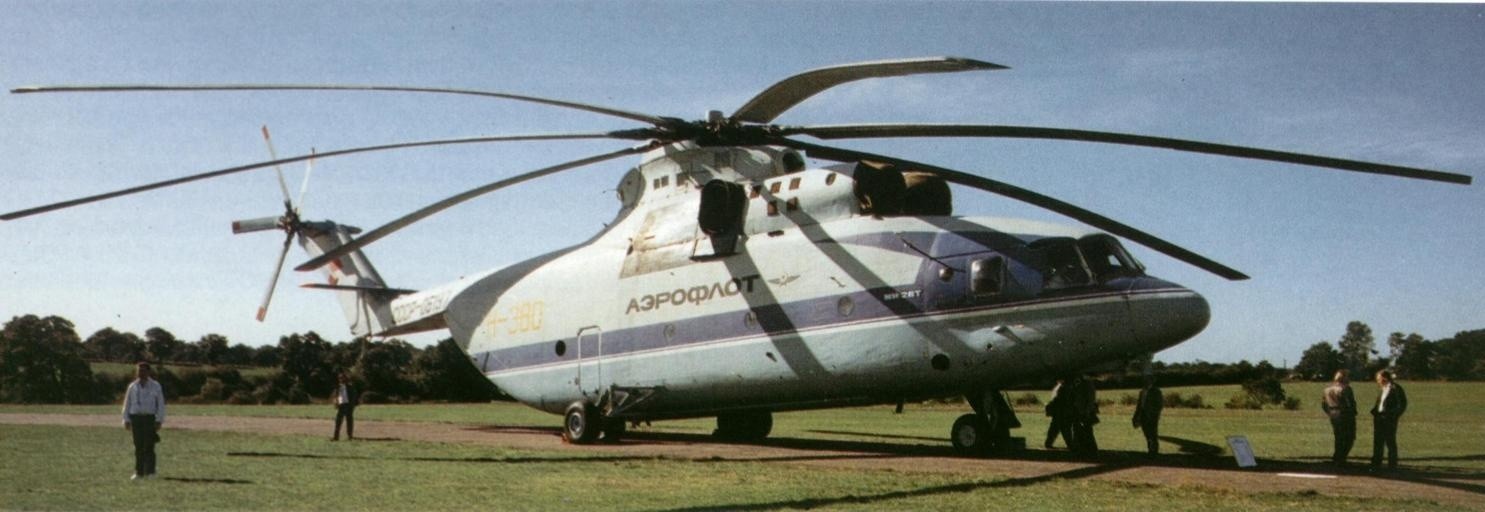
128;472;160;481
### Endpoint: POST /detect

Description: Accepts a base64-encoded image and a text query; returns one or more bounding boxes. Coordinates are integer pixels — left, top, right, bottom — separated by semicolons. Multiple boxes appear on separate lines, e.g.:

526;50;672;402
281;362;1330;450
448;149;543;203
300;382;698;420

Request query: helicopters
0;56;1472;458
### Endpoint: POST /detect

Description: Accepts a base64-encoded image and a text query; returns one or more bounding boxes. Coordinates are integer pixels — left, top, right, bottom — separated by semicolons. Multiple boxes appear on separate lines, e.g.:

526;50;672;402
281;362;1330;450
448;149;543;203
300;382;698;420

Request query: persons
1130;374;1163;456
121;360;168;481
1320;367;1360;472
325;371;360;441
1369;367;1408;471
1038;371;1099;453
